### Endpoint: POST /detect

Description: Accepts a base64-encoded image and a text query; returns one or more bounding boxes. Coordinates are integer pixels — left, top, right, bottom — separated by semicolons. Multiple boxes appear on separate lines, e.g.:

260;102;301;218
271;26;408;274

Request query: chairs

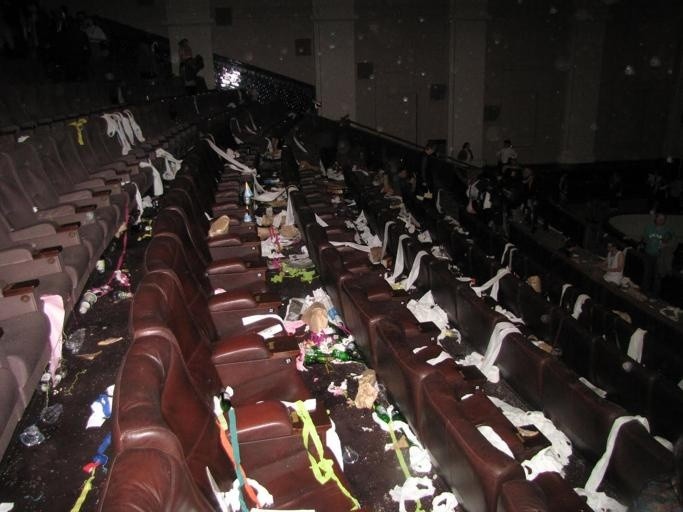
0;56;683;512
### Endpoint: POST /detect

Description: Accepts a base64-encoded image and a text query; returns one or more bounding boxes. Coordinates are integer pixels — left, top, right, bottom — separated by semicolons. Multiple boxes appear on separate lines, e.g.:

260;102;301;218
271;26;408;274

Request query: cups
79;291;97;315
342;444;360;466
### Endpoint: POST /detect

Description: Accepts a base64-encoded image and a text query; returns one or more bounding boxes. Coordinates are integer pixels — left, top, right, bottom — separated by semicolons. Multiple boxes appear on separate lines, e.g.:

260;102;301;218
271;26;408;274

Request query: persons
516;169;533;206
391;168;412;195
422;146;441;197
603;241;624;291
460;143;472;166
500;141;519;166
643;214;670;294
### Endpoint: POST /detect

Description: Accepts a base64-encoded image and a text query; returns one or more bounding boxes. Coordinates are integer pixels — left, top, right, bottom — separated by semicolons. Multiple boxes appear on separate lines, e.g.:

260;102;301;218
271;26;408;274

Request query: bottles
332;350;356;360
219;387;232;412
373;400;390;424
393;408;401;422
